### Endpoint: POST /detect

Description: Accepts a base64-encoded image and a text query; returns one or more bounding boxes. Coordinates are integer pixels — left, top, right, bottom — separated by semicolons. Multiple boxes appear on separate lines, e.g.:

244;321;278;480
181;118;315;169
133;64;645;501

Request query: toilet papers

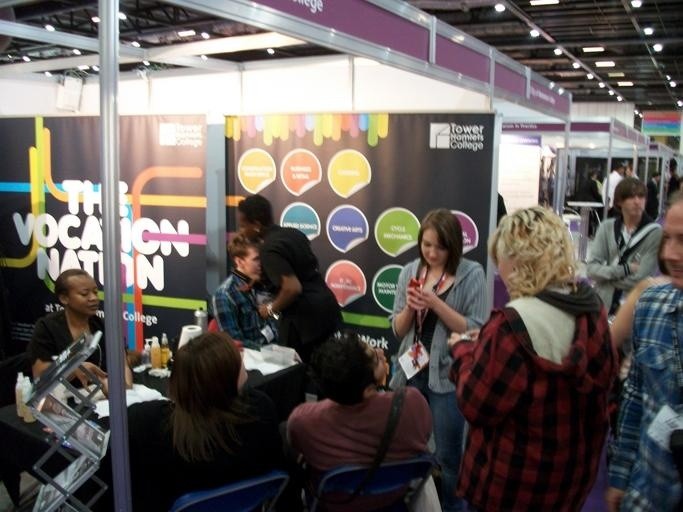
177;325;203;351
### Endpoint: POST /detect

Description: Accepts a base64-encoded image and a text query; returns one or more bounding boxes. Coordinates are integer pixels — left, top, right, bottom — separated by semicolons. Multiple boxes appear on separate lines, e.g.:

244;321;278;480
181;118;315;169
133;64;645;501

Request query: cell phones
411;278;420;301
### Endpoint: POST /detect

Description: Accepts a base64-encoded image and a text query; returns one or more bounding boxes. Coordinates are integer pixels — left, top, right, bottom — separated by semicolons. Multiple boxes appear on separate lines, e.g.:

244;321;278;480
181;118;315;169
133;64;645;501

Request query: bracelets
266;303;273;316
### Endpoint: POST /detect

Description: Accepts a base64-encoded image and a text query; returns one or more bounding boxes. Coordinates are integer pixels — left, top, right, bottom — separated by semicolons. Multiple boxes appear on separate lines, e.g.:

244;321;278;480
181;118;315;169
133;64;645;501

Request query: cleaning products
15;372;33;423
151;333;169;369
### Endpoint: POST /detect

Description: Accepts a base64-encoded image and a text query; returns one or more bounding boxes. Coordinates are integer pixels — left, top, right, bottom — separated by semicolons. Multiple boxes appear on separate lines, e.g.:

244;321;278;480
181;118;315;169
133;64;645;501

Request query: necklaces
81;326;90;337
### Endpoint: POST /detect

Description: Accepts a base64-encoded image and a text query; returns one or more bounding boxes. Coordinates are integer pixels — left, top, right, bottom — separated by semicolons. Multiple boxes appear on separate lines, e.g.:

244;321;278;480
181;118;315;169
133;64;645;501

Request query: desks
0;341;306;512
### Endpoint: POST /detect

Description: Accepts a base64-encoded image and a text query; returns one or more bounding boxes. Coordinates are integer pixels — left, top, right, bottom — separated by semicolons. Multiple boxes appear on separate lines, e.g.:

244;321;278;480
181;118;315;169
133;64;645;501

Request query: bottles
20;376;36;423
14;371;25;417
192;307;208;334
149;332;170;369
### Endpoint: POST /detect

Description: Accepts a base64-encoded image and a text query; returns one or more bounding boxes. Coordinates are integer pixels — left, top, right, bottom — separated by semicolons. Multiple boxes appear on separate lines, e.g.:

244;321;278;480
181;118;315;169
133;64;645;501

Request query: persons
237;193;343;355
27;269;132;398
389;207;489;511
607;230;674;385
283;338;433;511
446;207;619;512
586;177;662;439
129;333;278;511
607;190;682;512
583;158;683;241
213;228;280;352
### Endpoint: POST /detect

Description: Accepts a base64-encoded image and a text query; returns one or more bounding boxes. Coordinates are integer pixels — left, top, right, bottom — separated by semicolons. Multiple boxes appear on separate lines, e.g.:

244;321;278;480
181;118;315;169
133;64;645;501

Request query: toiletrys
140;338;154;366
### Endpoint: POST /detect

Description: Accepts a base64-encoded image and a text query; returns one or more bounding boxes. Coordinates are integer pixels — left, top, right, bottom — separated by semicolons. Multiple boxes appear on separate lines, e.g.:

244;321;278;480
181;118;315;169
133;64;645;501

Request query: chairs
0;352;34;405
311;455;438;511
172;470;293;512
